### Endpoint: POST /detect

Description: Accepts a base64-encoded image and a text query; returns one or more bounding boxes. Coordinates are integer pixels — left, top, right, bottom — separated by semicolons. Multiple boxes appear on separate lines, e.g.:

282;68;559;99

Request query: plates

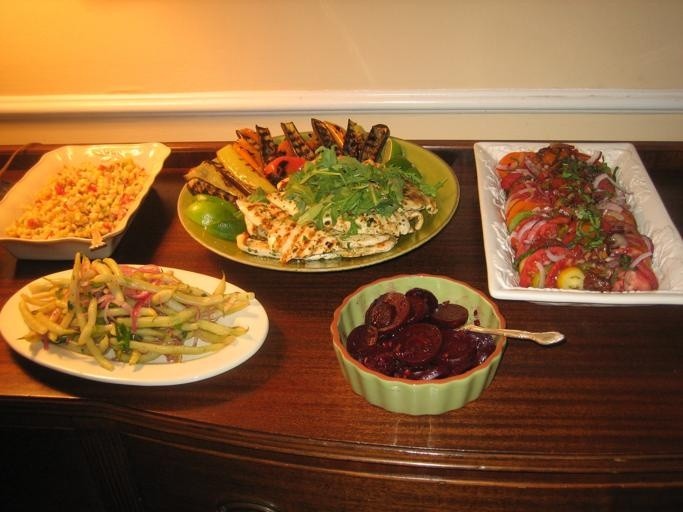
177;131;461;272
473;142;683;307
0;142;172;259
0;265;270;387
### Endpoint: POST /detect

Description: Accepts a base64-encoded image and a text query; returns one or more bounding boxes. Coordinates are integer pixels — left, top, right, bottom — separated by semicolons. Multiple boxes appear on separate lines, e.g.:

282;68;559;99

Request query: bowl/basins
330;273;509;417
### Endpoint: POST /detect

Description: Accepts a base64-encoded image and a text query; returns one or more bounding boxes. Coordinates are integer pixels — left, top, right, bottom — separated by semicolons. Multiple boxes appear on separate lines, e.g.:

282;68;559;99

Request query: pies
237;160;437;265
